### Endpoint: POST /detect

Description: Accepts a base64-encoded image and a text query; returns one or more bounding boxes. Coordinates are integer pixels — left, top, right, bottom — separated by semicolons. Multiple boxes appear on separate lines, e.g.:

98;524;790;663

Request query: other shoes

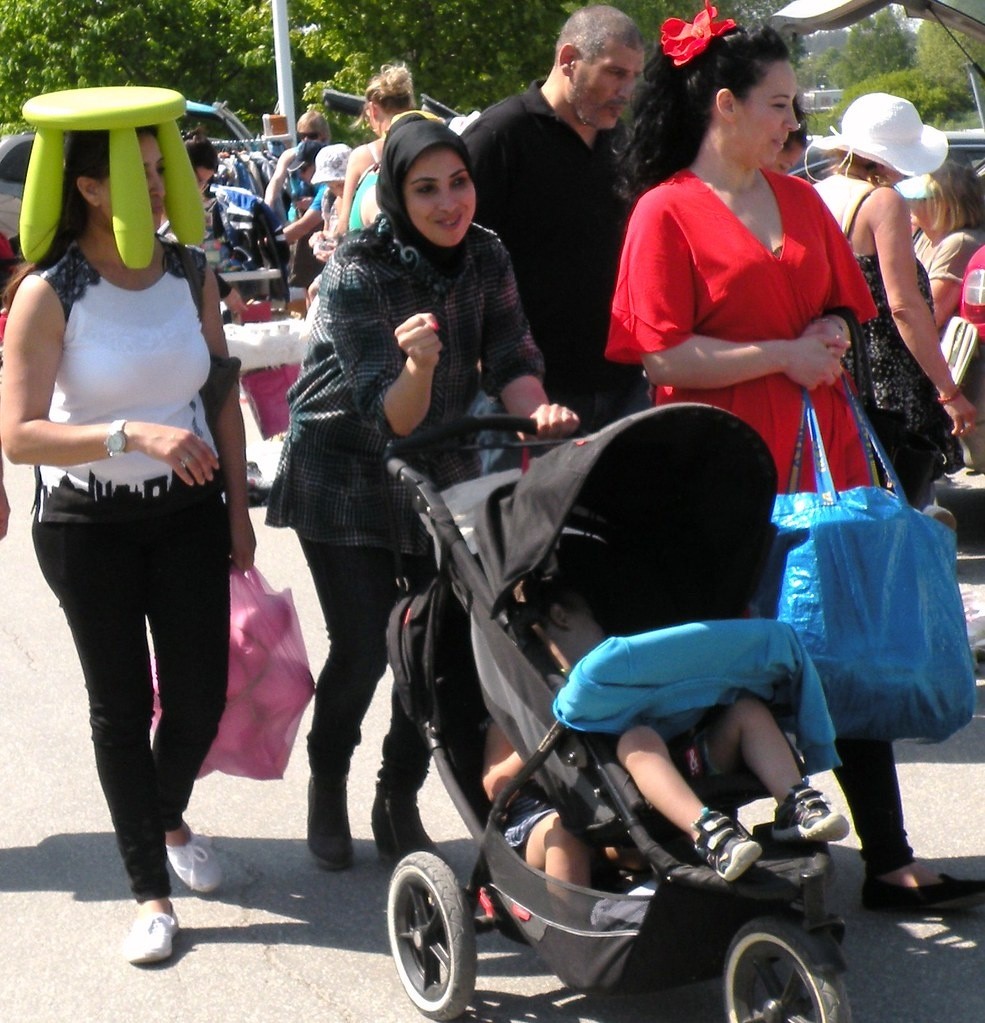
922;505;957;533
862;871;985;913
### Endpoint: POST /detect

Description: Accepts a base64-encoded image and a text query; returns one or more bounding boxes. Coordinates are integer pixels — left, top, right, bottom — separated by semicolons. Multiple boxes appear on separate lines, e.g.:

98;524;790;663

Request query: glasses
295;132;322;142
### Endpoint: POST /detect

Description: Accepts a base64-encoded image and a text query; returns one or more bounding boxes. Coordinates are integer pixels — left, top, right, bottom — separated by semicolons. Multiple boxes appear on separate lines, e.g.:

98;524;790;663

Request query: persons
264;7;852;919
1;120;258;967
607;23;984;919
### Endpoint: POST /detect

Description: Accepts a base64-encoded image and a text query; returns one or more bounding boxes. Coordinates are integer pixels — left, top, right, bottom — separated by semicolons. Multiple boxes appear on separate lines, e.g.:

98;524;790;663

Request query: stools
18;86;207;271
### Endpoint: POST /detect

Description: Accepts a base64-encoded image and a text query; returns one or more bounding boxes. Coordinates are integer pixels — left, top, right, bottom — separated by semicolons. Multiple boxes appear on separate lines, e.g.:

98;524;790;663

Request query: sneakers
126;902;179;964
165;819;222;893
770;784;850;842
693;806;762;881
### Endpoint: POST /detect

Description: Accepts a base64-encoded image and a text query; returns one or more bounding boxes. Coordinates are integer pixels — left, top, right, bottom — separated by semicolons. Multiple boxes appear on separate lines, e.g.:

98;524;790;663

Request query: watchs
104;419;129;458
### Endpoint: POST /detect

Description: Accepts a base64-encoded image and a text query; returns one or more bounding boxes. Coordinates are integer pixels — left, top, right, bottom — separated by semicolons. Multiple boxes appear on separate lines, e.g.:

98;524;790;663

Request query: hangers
213;137;265;160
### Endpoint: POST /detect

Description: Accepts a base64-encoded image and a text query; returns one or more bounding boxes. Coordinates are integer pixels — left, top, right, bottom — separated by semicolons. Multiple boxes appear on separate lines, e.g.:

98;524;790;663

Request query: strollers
384;400;856;1023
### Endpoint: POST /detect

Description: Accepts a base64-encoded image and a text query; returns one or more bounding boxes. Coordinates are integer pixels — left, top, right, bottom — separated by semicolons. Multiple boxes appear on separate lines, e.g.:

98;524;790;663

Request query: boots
307;769;353;870
371;783;457;879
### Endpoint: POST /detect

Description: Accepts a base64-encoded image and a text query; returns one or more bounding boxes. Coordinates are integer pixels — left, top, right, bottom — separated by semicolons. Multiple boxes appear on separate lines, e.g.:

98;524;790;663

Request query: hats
311;144;352;185
286;140;324;172
809;92;949;177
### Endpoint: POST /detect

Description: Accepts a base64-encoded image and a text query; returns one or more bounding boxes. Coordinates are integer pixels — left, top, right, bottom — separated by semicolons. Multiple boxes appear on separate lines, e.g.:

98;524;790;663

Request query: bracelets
937;388;962;405
812;316;848;358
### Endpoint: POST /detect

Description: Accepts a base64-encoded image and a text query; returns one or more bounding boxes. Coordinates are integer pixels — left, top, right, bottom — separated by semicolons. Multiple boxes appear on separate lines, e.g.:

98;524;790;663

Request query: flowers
660;0;736;67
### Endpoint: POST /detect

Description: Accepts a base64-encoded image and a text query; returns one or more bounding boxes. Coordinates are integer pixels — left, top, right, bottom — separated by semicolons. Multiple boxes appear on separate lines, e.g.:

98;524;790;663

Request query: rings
178;454;192;469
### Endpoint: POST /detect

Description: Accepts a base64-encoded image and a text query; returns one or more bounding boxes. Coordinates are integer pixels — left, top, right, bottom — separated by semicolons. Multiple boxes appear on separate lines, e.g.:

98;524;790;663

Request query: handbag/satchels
821;305;941;506
171;241;240;494
148;564;314;780
746;368;977;741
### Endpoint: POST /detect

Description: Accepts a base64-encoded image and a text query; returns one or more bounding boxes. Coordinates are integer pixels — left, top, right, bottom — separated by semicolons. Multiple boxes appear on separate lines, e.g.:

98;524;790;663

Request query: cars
767;0;985;202
